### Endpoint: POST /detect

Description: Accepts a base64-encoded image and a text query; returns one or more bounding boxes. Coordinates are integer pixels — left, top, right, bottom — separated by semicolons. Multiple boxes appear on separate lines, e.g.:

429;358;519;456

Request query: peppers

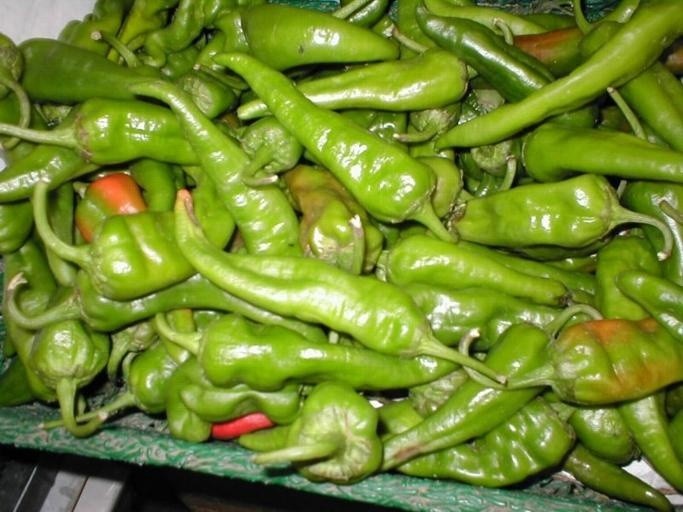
0;0;683;510
